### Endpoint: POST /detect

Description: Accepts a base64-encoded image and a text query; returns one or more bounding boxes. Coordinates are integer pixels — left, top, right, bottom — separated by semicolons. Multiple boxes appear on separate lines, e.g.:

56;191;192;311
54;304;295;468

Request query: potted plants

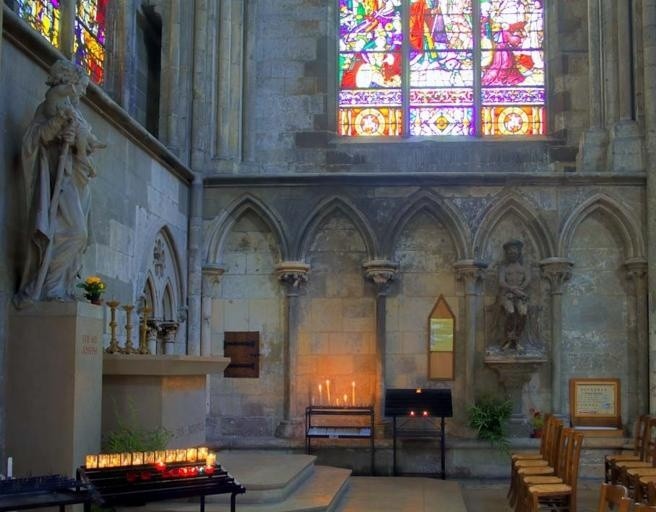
463;394;514;438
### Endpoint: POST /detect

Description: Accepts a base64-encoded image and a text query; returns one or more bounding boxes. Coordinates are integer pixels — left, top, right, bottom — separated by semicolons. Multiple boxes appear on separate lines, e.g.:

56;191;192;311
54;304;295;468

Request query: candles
318;380;355;405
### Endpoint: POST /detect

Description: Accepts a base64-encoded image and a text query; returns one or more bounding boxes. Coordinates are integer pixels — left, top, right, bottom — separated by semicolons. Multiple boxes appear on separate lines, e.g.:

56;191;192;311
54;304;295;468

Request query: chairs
507;413;656;511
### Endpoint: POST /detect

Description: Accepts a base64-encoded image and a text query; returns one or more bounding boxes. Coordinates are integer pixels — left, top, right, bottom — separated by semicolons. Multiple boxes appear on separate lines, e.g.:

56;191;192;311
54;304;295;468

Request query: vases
91;298;101;304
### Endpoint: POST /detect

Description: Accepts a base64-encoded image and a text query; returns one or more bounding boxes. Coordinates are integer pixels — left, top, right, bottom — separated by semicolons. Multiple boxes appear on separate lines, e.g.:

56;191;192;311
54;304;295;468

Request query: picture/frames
568;377;622;427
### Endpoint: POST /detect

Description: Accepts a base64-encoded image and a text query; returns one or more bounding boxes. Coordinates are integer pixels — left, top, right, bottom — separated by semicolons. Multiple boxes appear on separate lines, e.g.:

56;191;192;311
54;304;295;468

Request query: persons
5;61;95;310
494;241;534;352
42;59;109;170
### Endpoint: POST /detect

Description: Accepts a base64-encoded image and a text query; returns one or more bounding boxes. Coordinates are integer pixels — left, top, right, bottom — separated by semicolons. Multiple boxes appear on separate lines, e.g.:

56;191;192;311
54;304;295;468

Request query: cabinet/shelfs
306;406;375;475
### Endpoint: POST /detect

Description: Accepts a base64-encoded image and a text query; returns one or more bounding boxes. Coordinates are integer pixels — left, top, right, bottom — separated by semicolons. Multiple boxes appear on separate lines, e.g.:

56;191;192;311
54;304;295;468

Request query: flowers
76;273;105;300
